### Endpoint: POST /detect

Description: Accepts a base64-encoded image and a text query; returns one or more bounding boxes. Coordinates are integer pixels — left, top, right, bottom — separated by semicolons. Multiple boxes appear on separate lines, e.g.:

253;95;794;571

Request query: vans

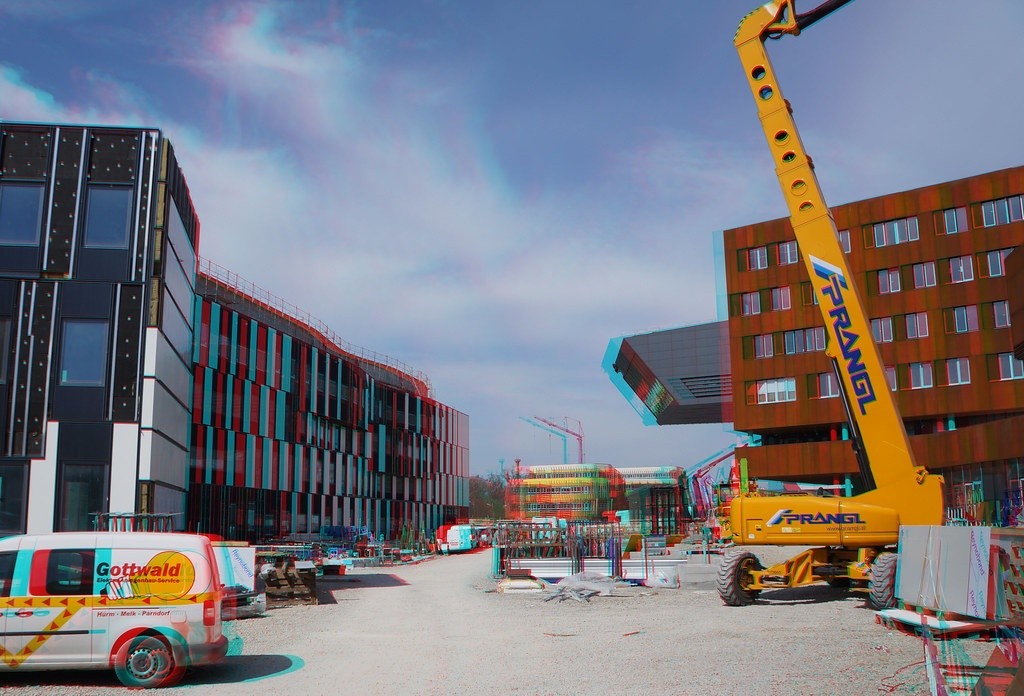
0;532;229;689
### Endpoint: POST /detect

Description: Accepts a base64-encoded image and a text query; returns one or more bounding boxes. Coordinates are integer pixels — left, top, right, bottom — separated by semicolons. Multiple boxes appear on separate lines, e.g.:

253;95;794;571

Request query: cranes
715;0;948;611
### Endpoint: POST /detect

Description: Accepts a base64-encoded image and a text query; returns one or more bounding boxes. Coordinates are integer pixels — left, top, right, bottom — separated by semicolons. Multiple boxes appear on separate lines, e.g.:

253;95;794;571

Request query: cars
446;524;495;551
254;551;359;581
